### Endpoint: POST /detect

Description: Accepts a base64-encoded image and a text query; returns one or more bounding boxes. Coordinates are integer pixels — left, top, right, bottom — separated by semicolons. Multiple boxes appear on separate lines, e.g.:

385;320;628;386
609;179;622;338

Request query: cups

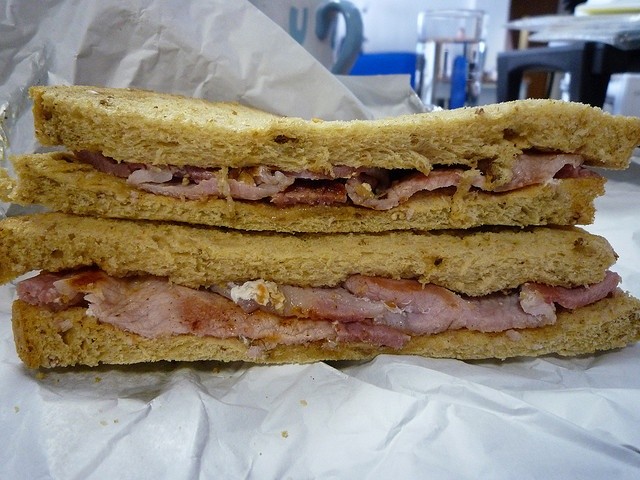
247;0;366;74
413;11;487;110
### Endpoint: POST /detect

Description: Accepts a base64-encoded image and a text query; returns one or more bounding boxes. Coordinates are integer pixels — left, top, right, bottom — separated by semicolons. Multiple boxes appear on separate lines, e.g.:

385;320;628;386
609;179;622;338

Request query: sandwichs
1;84;640;230
4;212;640;377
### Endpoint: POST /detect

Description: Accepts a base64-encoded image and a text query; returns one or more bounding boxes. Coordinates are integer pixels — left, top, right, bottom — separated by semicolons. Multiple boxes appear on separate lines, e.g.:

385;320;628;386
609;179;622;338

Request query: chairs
496;45;590;103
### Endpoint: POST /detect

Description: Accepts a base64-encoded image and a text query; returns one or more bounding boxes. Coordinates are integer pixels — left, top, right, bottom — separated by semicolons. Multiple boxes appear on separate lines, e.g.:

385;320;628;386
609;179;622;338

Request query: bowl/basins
347;51;422;95
